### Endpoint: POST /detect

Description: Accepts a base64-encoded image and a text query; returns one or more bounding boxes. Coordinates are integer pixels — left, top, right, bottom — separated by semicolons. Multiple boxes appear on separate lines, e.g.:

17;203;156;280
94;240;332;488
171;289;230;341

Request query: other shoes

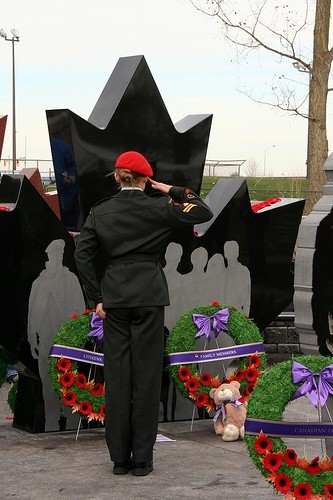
133;460;153;476
112;457;133;475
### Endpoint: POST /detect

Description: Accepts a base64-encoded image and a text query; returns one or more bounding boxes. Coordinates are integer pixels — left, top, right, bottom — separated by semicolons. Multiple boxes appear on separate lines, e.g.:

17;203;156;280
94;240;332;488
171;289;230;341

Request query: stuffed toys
209;381;249;442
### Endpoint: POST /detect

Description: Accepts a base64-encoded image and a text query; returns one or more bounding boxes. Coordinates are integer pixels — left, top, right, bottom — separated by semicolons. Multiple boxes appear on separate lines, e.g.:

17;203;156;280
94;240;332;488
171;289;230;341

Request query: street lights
0;27;20;174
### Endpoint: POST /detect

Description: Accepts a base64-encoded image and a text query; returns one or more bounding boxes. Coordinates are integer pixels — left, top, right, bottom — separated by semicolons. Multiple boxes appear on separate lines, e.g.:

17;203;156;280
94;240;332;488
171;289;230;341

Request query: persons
74;150;213;476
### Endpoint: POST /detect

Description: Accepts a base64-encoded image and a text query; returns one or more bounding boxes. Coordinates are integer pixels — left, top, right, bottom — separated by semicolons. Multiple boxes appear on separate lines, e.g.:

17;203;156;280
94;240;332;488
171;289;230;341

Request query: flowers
0;206;10;212
164;302;268;412
244;356;333;500
251;197;282;213
46;309;106;424
194;231;199;236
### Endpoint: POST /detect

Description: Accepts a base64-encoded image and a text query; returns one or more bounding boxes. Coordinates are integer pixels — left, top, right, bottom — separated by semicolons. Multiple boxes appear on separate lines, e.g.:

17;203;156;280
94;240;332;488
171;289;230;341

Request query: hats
114;150;154;177
